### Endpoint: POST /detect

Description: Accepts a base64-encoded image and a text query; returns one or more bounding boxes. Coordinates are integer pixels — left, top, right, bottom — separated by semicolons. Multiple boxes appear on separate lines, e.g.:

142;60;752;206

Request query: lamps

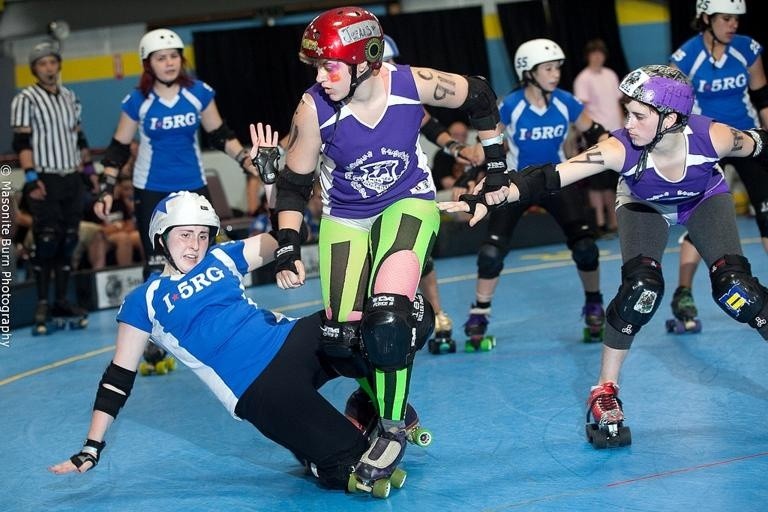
48;19;71;52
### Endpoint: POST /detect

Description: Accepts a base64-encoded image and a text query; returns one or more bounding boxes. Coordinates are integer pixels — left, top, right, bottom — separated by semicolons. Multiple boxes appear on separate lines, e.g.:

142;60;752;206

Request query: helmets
149;190;221;255
30;43;62;70
298;7;385;68
138;28;184;62
515;39;566;86
694;0;747;21
619;64;694;134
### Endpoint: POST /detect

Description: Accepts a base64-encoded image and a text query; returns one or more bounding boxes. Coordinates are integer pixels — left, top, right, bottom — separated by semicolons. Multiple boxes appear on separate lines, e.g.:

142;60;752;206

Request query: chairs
0;167;265;285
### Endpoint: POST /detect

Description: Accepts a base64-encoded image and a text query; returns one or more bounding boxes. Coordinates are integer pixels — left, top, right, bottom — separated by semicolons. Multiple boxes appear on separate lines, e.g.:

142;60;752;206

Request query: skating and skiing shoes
140;342;175;375
584;382;631;448
50;299;87;329
429;311;456;353
582;299;607;342
463;312;495;353
33;298;50;332
341;386;432;499
667;285;701;334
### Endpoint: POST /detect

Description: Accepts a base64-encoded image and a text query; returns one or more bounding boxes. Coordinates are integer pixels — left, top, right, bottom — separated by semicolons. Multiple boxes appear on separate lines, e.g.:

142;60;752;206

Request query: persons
419;120;509;203
47;122;436;491
451;38;613;336
93;28;259;363
668;1;768;320
9;42;102;336
574;38;625;235
1;122;323;285
437;63;768;425
276;34;478;336
274;6;498;482
